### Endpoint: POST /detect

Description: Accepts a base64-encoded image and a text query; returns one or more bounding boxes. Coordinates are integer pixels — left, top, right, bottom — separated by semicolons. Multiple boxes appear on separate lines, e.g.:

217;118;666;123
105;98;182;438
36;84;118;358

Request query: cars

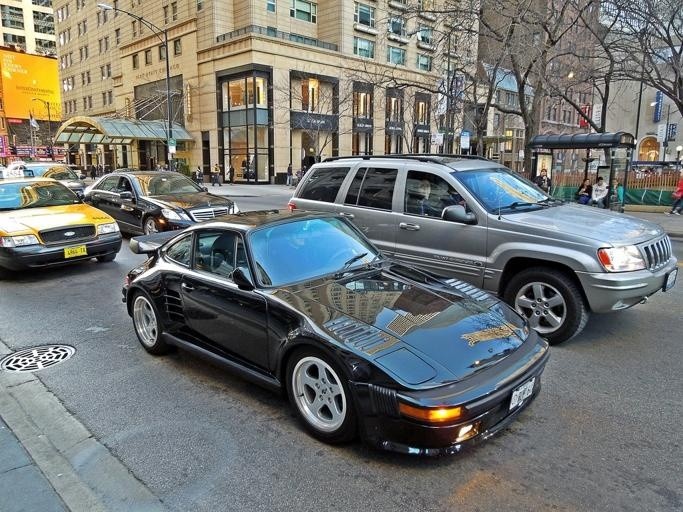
0;160;242;276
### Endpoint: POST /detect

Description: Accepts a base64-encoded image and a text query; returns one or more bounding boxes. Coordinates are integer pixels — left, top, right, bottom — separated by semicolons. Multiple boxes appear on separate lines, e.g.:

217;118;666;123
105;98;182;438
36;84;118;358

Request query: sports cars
121;205;555;467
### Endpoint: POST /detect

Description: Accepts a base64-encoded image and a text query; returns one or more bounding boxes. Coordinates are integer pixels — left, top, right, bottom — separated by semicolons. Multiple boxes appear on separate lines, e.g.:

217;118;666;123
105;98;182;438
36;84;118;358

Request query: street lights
675;145;683;173
649;101;671;169
31;98;52;157
96;2;172;171
567;70;591;180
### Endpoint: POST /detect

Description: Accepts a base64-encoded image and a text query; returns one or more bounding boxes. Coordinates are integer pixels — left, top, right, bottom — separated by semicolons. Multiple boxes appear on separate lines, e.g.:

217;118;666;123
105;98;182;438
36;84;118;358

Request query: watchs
89;164;96;180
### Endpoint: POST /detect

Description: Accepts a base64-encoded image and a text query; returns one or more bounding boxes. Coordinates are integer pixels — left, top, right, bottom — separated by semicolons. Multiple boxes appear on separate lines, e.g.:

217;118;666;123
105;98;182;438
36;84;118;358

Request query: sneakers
664;209;680;217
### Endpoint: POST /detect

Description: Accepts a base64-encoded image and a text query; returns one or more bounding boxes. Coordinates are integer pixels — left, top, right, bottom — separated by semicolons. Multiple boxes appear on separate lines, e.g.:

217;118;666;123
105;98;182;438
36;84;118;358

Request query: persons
406;176;446;218
212;164;222;186
670;173;683;215
285;163;292;186
302;164;309;176
226;164;233;184
532;169;552;195
587;177;609;208
439;184;467;222
576;179;591;204
195;166;203;184
608;178;623;206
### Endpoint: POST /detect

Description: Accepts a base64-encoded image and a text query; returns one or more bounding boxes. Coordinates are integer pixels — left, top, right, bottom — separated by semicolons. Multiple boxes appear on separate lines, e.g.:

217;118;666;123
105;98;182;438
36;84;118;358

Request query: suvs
285;152;681;347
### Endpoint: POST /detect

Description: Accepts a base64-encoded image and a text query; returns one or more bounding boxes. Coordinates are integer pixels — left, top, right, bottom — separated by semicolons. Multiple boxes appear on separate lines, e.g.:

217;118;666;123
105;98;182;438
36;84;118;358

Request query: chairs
210;232;248;277
